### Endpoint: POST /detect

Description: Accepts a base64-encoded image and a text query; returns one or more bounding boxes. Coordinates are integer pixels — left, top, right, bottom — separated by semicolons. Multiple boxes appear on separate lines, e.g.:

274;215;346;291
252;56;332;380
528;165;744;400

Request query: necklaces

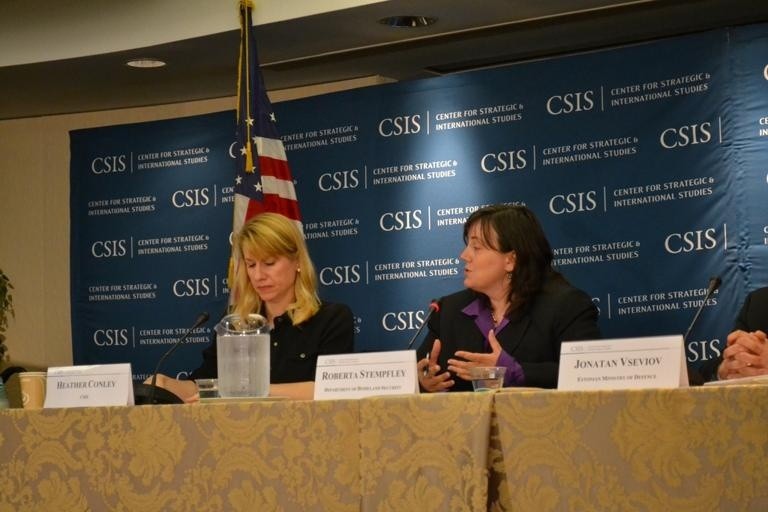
486;301;498;324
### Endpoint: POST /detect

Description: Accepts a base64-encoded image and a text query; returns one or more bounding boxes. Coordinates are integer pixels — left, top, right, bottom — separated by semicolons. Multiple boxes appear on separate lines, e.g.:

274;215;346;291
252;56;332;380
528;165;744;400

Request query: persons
715;285;767;380
143;211;354;403
416;204;598;391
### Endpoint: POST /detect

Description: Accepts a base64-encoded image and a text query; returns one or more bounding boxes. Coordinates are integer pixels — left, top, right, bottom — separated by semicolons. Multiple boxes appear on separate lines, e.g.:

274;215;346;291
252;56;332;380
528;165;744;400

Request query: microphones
408;299;443;349
133;311;210;404
683;275;721;342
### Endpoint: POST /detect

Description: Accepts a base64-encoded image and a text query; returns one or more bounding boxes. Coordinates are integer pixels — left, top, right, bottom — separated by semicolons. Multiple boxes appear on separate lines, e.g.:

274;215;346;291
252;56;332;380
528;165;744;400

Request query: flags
225;0;303;307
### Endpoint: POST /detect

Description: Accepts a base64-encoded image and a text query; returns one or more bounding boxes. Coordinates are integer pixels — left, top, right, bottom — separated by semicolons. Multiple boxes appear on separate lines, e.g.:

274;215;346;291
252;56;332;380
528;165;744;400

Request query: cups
214;314;272;397
19;371;47;409
195;378;218;403
467;367;507;393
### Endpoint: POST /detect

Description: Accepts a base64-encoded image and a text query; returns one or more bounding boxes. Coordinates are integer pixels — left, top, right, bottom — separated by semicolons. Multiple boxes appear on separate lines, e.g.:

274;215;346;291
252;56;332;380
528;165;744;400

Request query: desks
0;387;768;511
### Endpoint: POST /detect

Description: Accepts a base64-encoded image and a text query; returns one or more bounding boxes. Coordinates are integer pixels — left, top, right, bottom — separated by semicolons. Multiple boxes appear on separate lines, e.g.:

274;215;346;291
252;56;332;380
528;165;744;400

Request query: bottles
1;376;9;409
